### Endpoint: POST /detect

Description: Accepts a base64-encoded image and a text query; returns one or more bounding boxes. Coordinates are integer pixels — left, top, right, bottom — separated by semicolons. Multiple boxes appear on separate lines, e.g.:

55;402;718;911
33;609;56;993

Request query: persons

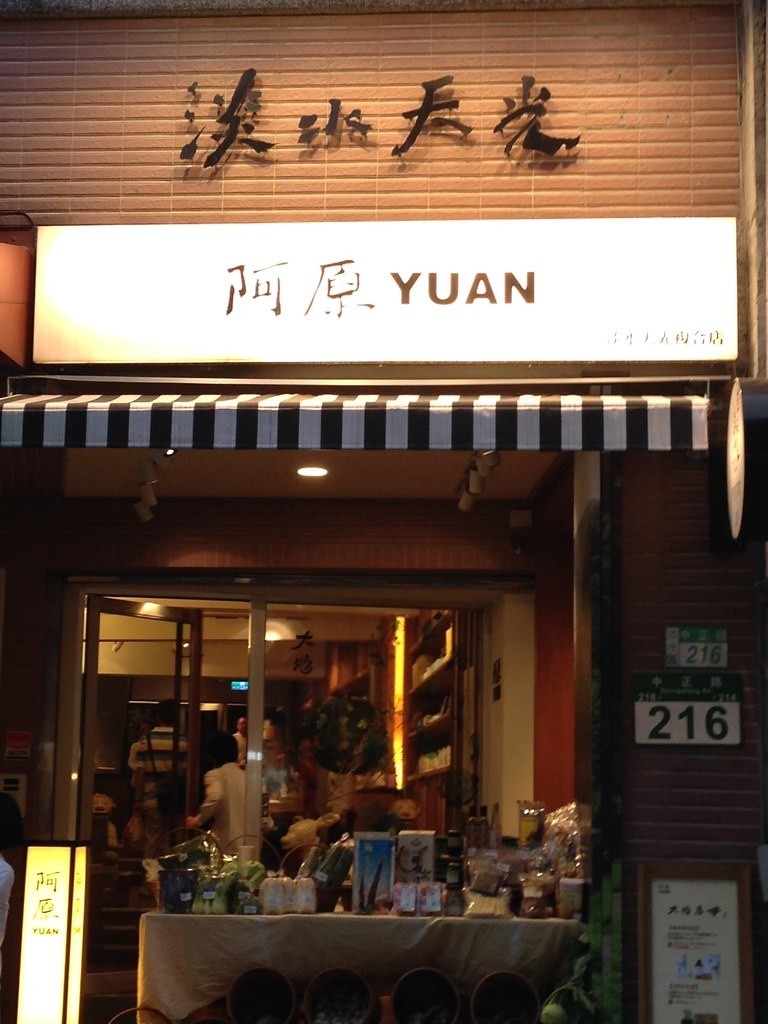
0;852;15;976
232;718;247;762
129;720;150;787
188;731;247;845
134;699;188;864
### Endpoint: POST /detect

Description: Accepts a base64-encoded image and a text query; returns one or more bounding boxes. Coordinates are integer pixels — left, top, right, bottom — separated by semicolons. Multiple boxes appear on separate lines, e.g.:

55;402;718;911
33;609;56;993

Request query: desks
136;909;585;1024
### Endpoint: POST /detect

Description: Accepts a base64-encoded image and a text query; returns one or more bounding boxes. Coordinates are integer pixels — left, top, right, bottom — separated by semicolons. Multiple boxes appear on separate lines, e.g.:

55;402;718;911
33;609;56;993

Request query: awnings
0;393;710;451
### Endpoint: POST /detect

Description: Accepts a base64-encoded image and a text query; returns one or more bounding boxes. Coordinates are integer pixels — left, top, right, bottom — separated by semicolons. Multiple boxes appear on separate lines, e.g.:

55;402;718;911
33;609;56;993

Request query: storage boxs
351;831;445;915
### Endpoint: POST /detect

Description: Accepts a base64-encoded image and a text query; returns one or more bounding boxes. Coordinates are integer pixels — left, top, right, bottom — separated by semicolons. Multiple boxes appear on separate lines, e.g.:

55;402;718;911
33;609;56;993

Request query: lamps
457;451;501;513
11;840;96;1024
112;642;123;652
134;456;159;523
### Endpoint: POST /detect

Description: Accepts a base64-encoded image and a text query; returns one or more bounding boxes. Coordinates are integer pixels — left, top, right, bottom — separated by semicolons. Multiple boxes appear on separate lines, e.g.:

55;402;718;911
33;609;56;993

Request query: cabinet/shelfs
401;606;467;782
